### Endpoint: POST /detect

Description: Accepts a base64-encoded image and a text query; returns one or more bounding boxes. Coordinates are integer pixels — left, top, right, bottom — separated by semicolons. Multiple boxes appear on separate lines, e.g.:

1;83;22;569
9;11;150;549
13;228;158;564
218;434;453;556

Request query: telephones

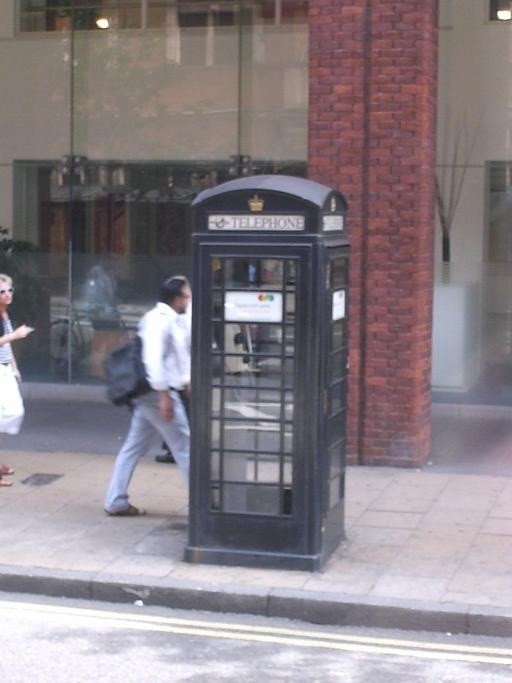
223;323;256;374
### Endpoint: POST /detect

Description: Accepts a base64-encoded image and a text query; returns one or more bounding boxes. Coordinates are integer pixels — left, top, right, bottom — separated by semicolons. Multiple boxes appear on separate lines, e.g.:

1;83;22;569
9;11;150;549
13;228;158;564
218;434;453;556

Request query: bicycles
48;303;131;372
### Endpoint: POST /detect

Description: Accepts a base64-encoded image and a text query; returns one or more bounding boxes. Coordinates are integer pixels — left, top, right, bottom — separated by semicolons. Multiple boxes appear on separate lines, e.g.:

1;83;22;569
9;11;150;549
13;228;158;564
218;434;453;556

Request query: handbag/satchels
99;338;151;406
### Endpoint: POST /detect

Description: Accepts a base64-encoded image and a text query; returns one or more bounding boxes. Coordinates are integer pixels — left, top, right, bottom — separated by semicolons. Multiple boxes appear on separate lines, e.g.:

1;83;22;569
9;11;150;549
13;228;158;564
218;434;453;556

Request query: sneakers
155;452;177;462
0;478;13;487
0;465;14;475
102;504;145;516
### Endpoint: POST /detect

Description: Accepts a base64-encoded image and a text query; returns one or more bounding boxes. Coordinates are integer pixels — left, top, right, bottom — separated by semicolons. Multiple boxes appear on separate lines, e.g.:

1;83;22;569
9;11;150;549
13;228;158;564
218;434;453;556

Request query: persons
103;275;192;516
151;276;192;463
1;272;35;486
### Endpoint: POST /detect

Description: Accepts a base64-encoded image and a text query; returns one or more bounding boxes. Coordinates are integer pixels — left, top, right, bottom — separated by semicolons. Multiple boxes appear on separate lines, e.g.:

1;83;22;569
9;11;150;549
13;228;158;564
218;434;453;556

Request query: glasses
0;288;14;294
